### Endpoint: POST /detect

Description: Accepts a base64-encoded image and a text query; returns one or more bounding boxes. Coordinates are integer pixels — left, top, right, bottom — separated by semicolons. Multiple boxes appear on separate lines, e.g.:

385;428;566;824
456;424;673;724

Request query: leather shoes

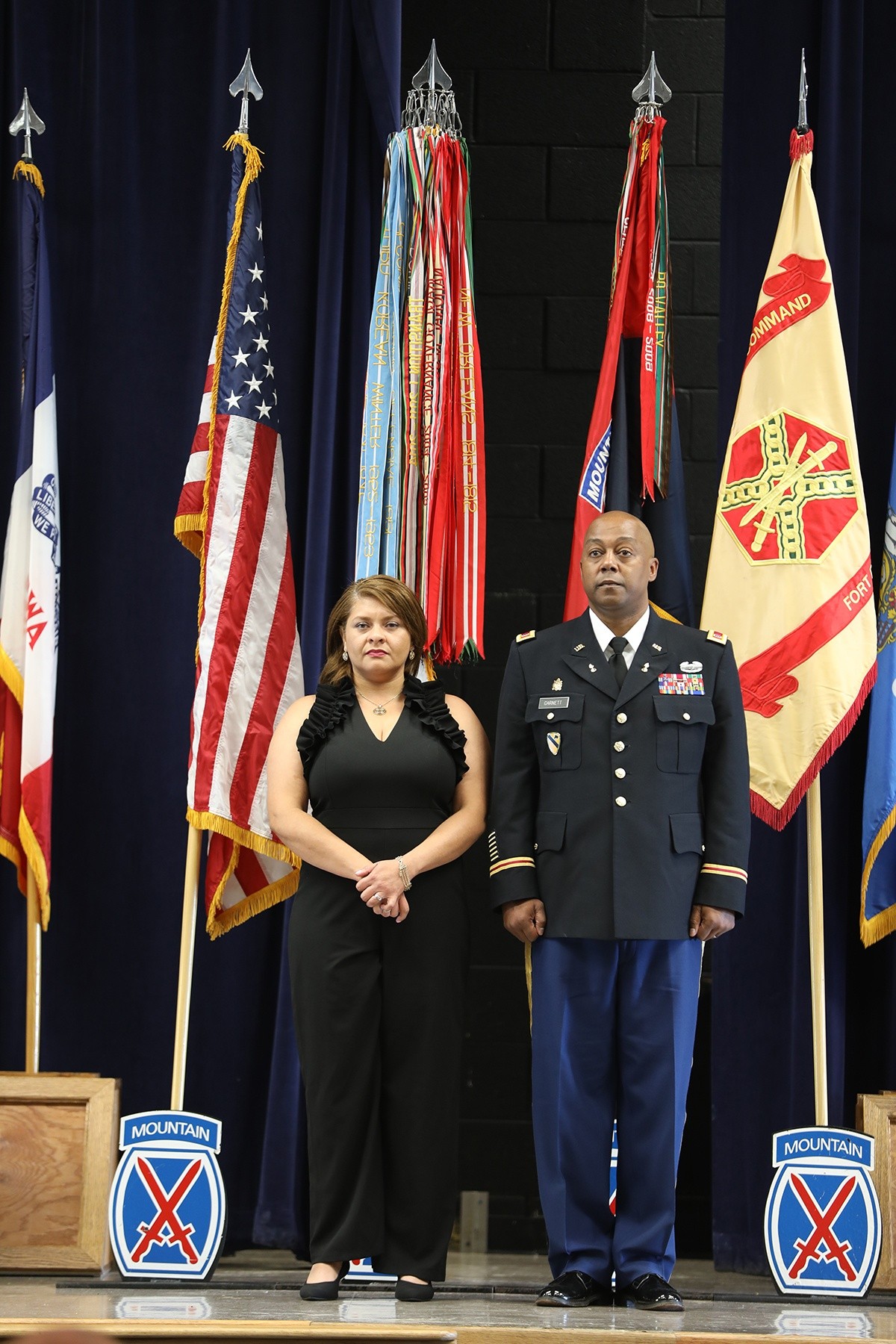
536;1269;613;1307
614;1273;684;1311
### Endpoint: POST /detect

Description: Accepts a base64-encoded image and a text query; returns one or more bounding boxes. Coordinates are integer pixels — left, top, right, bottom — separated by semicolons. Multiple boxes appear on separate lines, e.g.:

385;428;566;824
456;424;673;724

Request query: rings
374;894;382;901
714;935;717;938
382;910;391;912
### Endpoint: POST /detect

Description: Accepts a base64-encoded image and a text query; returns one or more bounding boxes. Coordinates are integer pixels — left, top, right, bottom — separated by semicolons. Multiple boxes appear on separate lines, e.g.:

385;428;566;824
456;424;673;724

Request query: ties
608;636;629;690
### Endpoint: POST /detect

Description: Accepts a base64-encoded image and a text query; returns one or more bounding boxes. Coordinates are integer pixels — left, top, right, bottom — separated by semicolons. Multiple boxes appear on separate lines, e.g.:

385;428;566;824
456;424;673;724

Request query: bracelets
395;856;412;891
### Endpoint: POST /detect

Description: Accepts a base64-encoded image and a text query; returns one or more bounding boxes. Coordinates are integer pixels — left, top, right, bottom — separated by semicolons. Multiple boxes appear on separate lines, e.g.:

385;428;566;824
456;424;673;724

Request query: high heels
394;1274;435;1301
300;1259;350;1300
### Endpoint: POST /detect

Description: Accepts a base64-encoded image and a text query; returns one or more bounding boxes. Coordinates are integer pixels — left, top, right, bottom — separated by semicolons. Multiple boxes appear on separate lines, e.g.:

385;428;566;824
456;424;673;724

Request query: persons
490;511;754;1310
266;577;489;1302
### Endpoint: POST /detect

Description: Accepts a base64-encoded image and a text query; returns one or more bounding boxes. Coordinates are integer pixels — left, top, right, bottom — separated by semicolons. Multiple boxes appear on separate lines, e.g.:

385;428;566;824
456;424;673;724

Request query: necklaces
354;686;403;715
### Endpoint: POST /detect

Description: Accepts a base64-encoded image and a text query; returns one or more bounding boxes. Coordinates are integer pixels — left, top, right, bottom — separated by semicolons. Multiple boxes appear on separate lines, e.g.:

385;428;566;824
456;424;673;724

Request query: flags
701;128;896;947
558;112;693;635
0;155;63;930
173;131;313;940
355;119;486;684
